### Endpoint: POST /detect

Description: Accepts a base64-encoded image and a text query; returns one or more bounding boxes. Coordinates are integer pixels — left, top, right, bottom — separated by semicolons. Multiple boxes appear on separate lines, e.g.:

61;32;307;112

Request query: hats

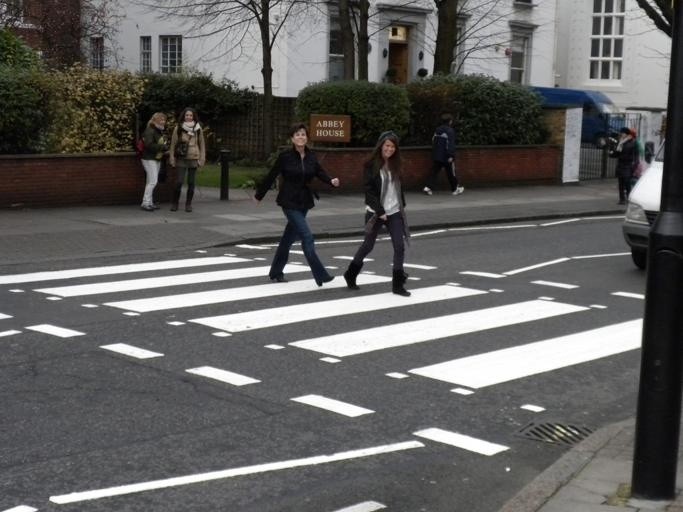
629;128;636;136
620;127;629;134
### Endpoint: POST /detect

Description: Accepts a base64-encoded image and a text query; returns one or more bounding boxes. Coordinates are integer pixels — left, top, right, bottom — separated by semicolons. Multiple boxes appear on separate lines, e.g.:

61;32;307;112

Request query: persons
139;112;170;210
419;113;465;196
629;127;645;179
170;108;206;212
343;130;412;297
252;124;341;287
609;127;635;204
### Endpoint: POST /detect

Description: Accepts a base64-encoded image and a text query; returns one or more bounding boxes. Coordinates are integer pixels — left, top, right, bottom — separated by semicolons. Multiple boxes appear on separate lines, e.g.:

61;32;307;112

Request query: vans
621;135;665;273
526;86;625;150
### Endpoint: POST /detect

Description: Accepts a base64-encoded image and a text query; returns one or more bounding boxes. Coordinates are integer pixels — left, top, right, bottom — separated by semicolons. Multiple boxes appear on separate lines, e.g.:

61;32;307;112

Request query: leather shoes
269;271;287;282
316;275;334;287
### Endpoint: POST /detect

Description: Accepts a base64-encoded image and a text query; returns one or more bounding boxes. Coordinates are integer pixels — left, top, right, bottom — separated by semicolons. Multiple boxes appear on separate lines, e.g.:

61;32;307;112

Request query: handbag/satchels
174;142;188;158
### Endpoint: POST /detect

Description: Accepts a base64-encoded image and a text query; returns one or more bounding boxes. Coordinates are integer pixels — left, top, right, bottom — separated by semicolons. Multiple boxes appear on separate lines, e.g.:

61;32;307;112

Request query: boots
392;269;410;296
344;261;363;290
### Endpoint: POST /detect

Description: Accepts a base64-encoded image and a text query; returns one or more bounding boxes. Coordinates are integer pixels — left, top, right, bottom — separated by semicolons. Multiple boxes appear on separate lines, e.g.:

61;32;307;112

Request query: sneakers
185;205;192;212
423;186;432;196
170;204;177;211
149;204;156;208
140;204;153;211
452;185;464;195
